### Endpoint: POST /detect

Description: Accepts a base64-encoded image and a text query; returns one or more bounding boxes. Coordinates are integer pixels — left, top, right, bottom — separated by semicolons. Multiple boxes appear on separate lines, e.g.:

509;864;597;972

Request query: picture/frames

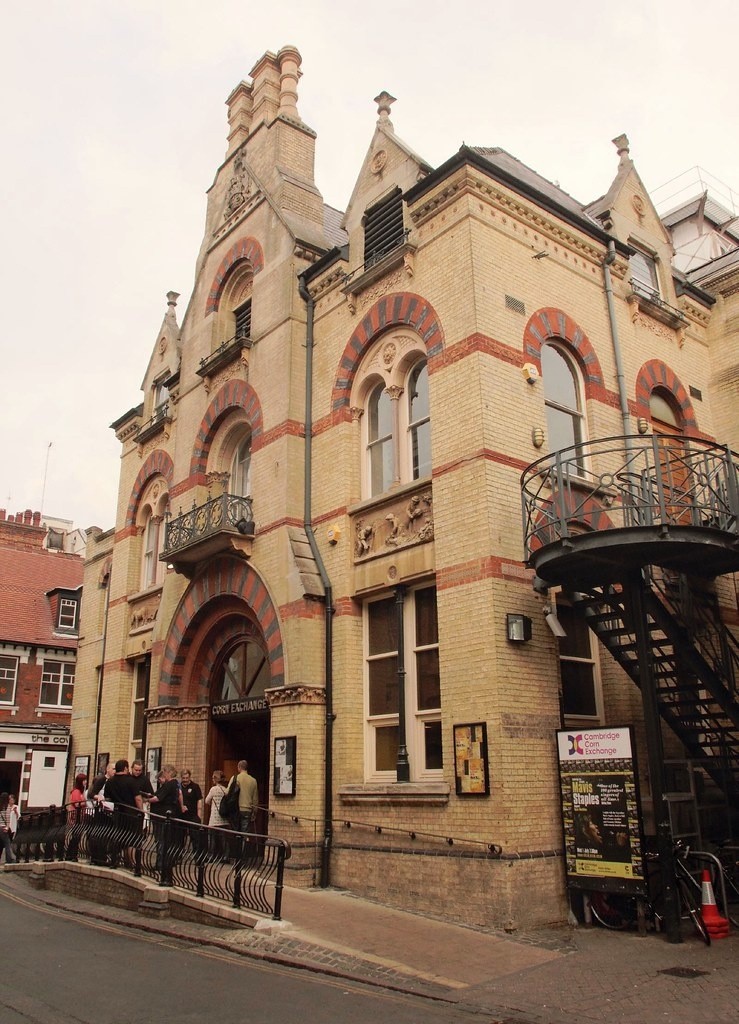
273;735;297;795
452;721;491;797
146;746;162;792
97;752;110;776
73;754;90;790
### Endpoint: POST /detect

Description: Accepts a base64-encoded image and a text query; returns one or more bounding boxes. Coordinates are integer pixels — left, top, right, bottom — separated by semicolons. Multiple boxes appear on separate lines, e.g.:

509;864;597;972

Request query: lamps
506;612;532;643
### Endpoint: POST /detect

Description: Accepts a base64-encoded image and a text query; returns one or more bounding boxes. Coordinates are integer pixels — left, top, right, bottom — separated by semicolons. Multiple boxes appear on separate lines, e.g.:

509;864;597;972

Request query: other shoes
206;857;213;863
219;859;228;863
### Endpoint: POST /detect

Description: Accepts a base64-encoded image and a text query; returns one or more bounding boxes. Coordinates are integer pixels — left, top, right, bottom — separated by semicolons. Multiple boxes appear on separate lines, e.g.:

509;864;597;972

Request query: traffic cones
697;870;729;938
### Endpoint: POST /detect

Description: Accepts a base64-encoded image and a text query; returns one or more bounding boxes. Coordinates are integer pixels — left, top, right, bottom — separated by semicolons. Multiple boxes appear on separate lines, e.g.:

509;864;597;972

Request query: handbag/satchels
219;782;240;816
8;828;11;836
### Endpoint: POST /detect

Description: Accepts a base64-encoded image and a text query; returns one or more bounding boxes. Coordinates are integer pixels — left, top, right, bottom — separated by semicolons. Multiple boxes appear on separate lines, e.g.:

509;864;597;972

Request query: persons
279;741;286;755
205;770;231;863
83;759;181;872
64;773;88;862
287;765;292;781
574;809;631;863
8;794;24;860
219;760;260;865
177;768;207;862
0;792;16;863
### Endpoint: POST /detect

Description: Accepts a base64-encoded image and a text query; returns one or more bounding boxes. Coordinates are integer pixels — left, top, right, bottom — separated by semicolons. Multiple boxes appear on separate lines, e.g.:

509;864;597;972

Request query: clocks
369;149;388;173
230;192;245;209
631;194;647;216
157;336;168;355
